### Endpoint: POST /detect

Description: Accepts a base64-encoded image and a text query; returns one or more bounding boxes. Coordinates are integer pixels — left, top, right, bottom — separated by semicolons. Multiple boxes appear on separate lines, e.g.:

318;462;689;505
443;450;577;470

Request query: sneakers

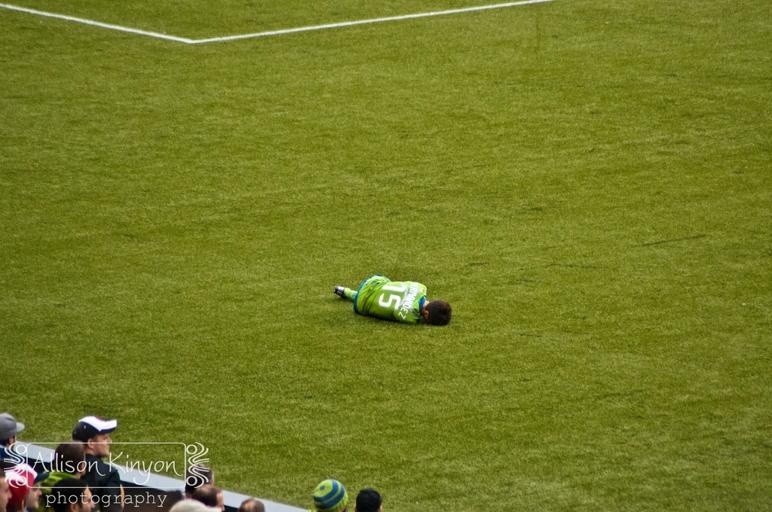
334;286;348;299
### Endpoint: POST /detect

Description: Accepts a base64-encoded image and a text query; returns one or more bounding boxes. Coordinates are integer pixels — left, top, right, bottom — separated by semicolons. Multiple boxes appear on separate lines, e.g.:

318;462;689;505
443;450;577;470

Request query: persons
0;413;383;512
333;274;452;326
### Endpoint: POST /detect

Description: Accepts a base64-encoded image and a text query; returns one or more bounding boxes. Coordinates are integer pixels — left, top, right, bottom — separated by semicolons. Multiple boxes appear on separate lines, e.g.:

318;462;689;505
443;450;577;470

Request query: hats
313;479;348;512
4;462;49;505
0;413;24;441
72;415;118;441
